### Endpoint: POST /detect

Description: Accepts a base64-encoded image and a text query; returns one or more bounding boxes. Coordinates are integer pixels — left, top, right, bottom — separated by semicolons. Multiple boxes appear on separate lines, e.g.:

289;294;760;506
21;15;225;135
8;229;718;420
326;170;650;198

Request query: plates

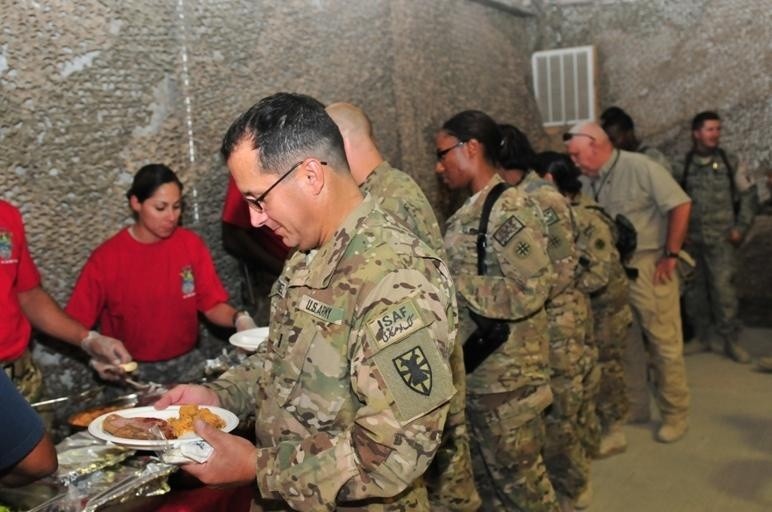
88;403;240;452
228;326;272;353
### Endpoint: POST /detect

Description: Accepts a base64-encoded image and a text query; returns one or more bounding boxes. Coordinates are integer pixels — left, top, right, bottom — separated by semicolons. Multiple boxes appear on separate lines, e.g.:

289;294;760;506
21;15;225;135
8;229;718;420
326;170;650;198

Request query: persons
667;111;754;363
0;90;480;512
435;107;693;512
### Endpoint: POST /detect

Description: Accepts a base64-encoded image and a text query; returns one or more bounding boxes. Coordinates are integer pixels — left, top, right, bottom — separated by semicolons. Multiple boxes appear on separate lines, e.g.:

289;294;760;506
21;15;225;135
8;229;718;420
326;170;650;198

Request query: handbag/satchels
613;214;639;263
458;306;510;373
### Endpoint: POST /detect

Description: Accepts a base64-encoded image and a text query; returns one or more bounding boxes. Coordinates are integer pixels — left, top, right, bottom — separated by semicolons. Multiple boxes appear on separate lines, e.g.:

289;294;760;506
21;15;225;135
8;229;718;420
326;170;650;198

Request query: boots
684;333;712;354
725;332;751;363
599;419;627;456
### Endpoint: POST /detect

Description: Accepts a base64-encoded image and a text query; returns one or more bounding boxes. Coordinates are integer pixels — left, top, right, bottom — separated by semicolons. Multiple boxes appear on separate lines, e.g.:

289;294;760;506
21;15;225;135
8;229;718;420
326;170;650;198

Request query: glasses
563;133;595;142
235;158;328;214
436;136;479;160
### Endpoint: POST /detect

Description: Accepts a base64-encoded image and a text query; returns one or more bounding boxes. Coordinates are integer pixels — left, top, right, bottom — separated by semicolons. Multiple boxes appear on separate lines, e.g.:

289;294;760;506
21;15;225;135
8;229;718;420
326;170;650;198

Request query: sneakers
759;356;772;371
657;413;689;442
